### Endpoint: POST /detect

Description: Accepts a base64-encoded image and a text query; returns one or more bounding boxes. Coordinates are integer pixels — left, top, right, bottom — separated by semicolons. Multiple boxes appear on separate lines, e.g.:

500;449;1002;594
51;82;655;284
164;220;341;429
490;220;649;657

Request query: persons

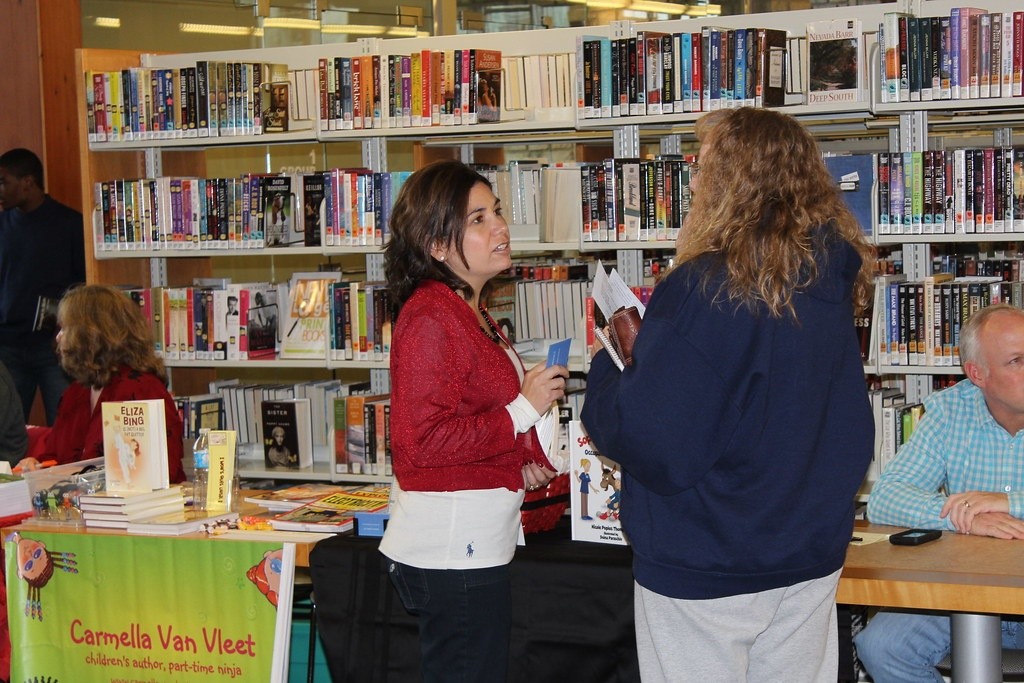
0;148;85;431
266;427;292;468
0;361;29;470
377;159;570;683
226;295;239;316
579;109;876;683
481;79;498;115
36;285;187;485
853;304;1024;683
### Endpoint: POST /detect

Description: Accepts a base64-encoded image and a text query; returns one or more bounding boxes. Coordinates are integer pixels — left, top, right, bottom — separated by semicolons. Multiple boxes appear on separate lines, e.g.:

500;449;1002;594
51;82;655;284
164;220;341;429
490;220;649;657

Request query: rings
961;501;971;508
528;484;535;492
535;481;541;490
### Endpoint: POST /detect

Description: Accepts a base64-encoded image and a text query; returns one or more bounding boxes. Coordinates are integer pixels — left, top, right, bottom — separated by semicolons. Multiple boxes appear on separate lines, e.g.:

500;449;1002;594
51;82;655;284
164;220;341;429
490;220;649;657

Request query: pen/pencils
852;536;864;541
12;460;57;471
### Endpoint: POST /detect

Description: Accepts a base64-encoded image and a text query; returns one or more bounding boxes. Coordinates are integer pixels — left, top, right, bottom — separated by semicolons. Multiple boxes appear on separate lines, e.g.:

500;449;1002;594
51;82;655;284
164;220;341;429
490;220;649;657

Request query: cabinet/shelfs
74;0;1024;485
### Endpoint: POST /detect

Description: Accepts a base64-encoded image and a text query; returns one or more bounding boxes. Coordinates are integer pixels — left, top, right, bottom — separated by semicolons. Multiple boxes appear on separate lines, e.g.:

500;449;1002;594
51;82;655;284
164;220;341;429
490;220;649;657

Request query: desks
0;489;1024;683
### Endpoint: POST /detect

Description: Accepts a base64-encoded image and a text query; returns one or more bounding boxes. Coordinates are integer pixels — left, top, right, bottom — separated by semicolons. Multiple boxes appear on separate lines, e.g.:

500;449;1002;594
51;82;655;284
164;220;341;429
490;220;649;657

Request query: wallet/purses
607;306;643;366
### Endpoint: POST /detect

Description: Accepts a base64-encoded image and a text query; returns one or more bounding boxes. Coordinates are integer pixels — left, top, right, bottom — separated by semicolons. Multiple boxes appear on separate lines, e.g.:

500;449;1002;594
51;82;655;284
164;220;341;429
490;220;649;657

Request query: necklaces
476;306;500;343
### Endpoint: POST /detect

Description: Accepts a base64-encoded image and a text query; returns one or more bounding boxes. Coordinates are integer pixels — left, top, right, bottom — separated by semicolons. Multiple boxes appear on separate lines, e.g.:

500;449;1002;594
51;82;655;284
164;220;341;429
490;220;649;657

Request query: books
0;7;1024;539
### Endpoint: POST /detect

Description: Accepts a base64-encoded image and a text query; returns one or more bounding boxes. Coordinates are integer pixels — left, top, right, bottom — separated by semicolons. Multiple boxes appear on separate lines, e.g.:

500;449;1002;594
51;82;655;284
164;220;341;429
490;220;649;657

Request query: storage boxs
21;456;105;528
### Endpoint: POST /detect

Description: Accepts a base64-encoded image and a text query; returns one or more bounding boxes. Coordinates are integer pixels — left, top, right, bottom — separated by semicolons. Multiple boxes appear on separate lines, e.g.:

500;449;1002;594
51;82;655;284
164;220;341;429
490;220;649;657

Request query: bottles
193;428;210;510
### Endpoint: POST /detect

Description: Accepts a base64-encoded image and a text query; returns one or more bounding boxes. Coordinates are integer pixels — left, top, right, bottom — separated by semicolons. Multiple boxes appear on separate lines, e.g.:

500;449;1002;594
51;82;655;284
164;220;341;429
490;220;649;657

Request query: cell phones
890;529;942;546
